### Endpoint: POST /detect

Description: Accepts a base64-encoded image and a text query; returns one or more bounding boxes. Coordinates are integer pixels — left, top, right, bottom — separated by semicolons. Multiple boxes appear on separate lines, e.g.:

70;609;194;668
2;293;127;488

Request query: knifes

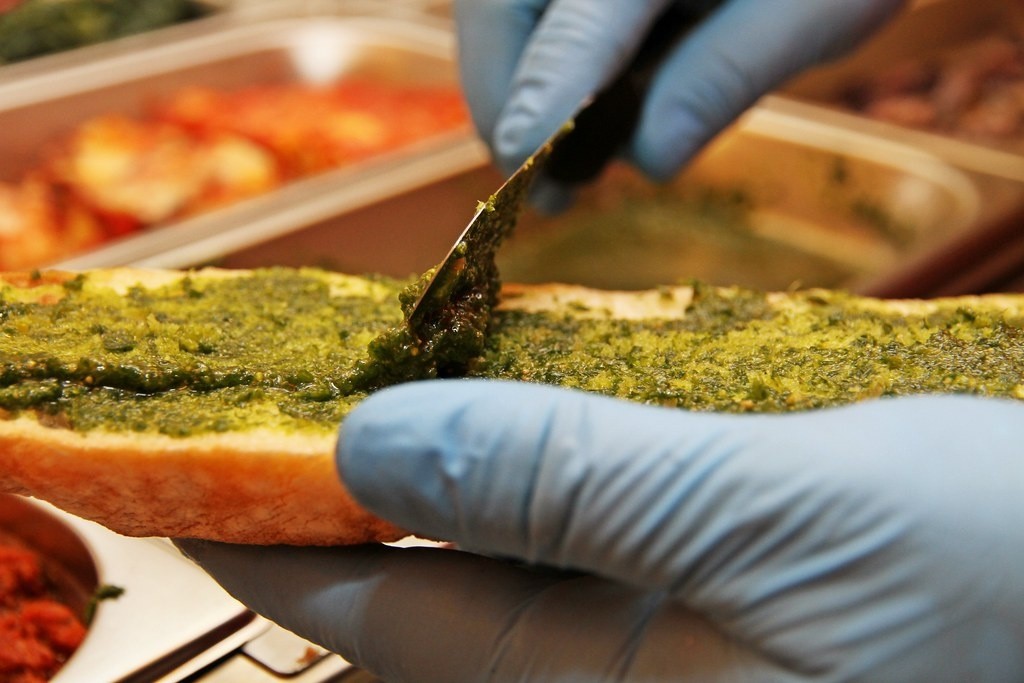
405;0;731;337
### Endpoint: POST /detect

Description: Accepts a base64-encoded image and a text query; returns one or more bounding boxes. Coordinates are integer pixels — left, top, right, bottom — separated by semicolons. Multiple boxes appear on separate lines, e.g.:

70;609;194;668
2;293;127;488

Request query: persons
171;0;1024;683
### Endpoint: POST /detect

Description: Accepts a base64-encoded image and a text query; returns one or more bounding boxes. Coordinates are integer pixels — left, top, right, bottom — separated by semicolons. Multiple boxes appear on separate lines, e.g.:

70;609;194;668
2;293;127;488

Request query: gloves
454;0;905;219
170;377;1024;683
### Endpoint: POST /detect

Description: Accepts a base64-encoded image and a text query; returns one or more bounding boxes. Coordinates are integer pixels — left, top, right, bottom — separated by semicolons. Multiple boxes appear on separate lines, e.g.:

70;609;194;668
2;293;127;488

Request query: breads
1;268;1024;546
